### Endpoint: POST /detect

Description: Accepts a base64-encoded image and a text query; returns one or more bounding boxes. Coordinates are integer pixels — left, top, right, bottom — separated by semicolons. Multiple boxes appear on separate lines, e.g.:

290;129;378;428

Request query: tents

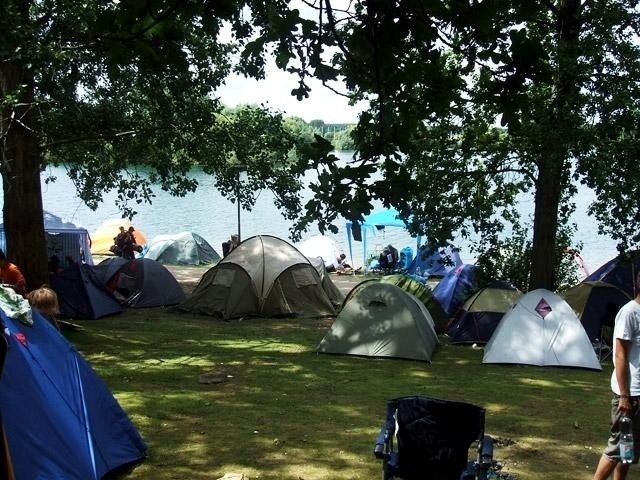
0;282;150;480
136;232;222;266
171;233;338;321
48;254;58;279
305;256;345;305
51;259;124;322
581;247;640;299
559;280;634;351
89;219;146;256
312;282;441;367
93;256;186;308
431;263;514;317
1;208;94;273
441;278;525;346
407;240;464;282
339;274;450;334
480;288;604;372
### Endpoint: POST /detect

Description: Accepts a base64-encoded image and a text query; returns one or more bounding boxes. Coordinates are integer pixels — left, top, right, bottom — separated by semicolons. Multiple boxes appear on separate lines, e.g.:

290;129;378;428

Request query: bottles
617;415;633;465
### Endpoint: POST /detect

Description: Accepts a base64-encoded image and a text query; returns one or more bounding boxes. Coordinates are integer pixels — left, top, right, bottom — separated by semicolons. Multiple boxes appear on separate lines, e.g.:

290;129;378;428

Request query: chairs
593;325;613;363
374;396;493;480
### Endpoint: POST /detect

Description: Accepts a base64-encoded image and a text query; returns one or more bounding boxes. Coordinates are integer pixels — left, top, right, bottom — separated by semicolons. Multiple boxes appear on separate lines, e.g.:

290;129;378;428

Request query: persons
25;283;61;332
225;234;239;257
0;247;28;297
592;268;640;479
119;227;136;259
334;243;398;273
117;226;123;258
